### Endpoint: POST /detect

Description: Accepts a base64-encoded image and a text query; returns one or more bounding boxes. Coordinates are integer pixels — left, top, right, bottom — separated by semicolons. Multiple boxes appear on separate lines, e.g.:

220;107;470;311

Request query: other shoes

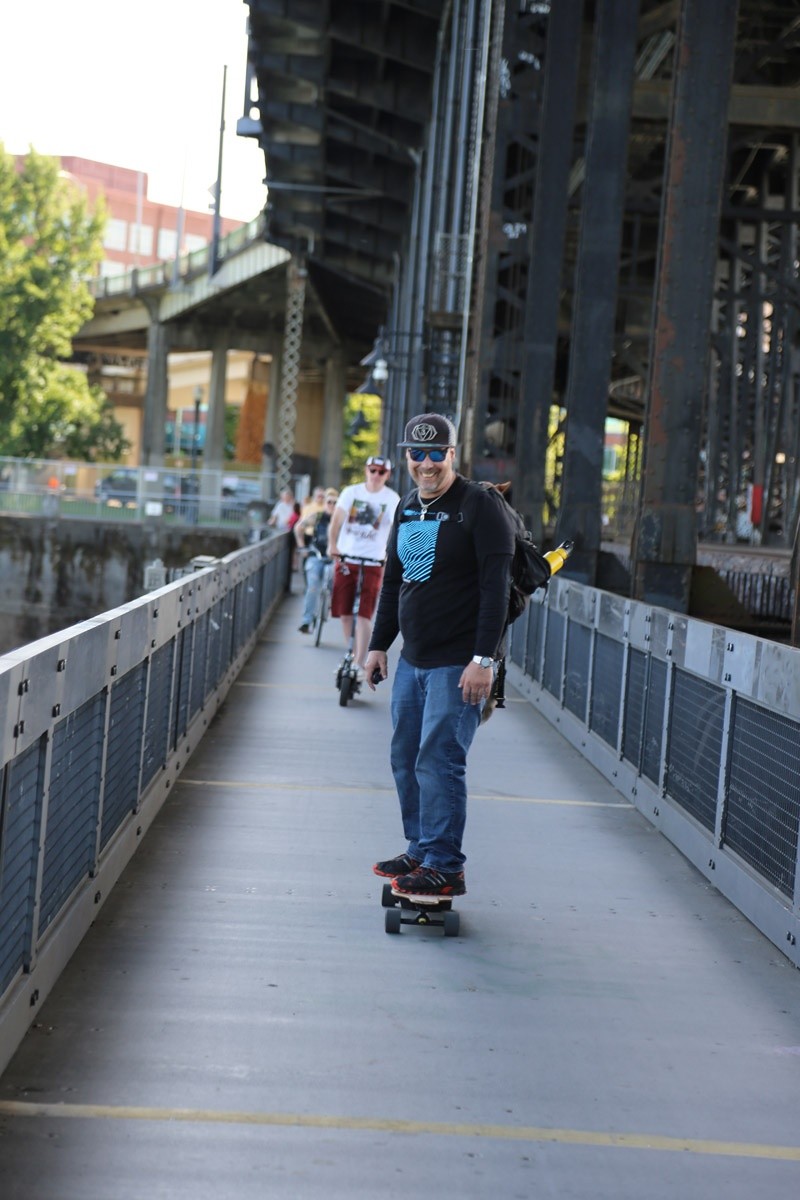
300;625;308;632
351;663;364;681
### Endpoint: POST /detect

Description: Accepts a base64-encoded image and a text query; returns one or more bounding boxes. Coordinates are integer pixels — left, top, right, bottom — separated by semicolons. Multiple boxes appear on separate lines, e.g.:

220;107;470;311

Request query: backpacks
399;478;551;624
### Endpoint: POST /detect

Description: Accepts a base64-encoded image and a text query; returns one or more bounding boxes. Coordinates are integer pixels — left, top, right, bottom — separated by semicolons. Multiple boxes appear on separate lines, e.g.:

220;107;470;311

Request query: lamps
356;371;380;394
349;404;369;428
359;325;401;380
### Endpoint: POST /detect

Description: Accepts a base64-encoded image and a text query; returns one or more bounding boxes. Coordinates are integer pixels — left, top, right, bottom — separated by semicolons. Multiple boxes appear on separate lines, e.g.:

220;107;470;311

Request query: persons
328;457;402;683
301;488;325;593
362;412;551;900
268;488;297;573
294;487;337;634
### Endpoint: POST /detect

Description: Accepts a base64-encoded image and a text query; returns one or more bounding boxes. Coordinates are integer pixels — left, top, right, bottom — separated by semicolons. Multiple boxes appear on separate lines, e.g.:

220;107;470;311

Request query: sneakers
372;853;420;878
390;866;467;896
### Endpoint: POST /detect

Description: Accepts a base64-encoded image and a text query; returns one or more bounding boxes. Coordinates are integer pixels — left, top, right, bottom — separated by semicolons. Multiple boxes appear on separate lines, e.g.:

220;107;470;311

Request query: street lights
187;384;204;521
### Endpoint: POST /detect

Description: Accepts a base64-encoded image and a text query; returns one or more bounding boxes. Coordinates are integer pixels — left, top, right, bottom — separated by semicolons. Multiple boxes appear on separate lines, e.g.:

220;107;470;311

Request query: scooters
329;553;387;706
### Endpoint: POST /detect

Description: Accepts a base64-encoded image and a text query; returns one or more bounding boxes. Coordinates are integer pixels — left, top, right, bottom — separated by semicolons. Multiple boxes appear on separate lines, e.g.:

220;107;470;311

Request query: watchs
473;655;494;668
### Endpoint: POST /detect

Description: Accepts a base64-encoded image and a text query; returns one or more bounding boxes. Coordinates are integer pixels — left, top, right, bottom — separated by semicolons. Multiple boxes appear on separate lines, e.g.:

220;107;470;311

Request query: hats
397;413;456;447
367;454;392;470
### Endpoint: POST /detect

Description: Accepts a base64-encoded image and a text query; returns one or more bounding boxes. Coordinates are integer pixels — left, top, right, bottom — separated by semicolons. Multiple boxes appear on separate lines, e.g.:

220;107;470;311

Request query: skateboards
381;877;460;937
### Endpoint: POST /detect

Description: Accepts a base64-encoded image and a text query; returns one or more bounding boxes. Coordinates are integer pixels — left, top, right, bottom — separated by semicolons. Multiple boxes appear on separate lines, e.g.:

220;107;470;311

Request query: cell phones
372;667;381;684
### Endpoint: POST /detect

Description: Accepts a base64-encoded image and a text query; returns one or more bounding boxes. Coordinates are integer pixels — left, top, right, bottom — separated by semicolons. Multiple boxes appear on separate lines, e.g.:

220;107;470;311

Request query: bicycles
296;544;337;646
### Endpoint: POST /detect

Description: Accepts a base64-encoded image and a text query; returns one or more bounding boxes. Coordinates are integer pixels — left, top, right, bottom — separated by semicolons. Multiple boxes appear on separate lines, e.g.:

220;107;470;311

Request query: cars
92;468;277;522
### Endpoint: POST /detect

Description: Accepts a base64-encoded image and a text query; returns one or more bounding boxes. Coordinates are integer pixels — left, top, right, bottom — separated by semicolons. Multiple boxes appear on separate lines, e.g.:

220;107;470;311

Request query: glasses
407;448;449;462
368;468;385;475
327;500;336;504
